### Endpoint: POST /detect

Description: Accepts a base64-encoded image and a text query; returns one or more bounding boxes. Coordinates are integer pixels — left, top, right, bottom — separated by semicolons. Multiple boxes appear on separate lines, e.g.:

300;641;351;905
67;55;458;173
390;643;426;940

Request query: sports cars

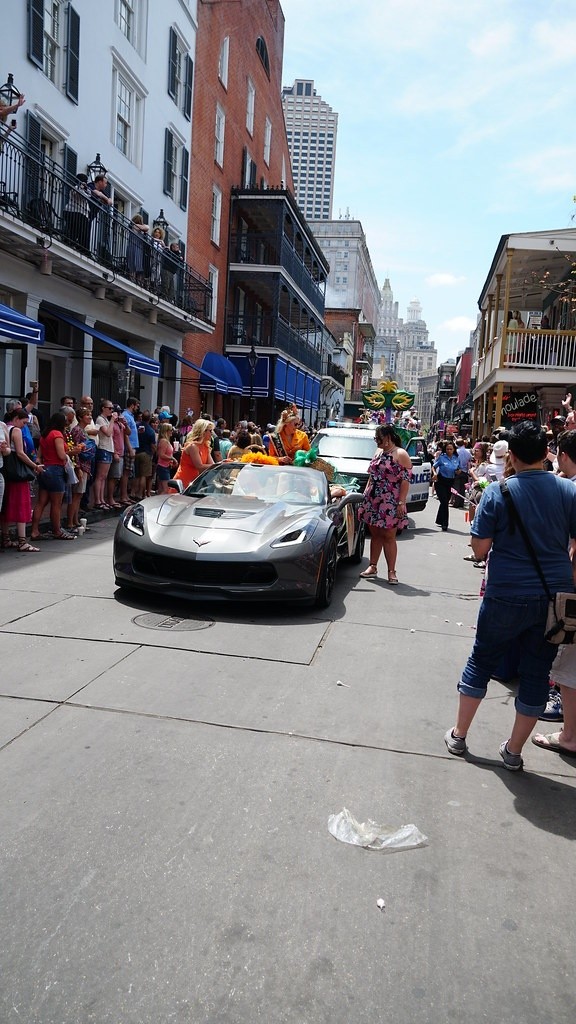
113;460;364;613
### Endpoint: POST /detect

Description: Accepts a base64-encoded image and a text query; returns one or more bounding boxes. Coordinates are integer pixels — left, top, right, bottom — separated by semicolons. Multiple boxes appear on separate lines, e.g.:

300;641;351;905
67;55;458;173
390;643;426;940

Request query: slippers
532;731;576;757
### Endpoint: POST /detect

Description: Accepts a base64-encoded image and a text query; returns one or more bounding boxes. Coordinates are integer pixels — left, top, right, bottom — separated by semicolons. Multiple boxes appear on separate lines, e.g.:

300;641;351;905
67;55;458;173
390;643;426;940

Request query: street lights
335;398;341;428
247;344;259;423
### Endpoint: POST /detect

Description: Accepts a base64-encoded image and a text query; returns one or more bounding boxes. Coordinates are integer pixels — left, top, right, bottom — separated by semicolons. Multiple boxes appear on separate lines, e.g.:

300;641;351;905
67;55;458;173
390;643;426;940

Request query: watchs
399;500;403;506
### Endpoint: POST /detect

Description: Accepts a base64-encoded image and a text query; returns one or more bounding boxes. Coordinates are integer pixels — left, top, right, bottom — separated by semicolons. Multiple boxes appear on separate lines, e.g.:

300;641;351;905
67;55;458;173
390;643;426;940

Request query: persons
504;311;552;369
0;384;317;552
357;424;413;585
427;394;576;771
359;408;416;428
128;215;183;301
63;174;112;259
0;93;26;143
309;477;346;499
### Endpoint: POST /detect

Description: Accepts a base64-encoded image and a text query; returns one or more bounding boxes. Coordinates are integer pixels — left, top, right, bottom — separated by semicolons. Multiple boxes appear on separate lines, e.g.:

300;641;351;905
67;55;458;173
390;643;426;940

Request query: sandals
0;496;137;552
360;564;377;577
388;570;398;584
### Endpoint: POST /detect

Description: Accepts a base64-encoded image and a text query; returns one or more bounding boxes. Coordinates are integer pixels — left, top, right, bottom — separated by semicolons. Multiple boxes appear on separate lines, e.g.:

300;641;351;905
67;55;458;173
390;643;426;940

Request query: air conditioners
361;375;368;387
363;343;372;354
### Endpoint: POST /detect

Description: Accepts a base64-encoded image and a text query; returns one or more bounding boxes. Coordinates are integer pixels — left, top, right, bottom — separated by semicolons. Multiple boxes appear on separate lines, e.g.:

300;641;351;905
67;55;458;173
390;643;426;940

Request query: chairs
113;257;130;271
28;197;65;235
0;180;19;218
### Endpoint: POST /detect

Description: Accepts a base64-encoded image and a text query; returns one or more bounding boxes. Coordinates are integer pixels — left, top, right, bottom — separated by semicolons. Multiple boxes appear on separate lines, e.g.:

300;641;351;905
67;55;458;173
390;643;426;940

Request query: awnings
161;347;228;394
0;302;45;346
200;352;243;396
37;306;161;378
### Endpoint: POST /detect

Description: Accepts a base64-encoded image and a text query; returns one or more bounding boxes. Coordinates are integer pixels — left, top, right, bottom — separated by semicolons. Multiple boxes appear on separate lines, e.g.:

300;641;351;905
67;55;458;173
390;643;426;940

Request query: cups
173;442;179;451
77;527;84;535
80;519;87;529
139;426;144;433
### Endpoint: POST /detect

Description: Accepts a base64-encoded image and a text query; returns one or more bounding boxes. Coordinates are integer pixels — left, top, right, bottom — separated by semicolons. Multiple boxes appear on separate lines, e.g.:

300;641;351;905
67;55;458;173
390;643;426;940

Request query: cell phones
11;119;16;128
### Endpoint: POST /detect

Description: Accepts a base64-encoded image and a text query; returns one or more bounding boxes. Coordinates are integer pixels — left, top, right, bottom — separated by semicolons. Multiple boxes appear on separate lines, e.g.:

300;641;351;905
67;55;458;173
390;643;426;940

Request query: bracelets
33;391;38;393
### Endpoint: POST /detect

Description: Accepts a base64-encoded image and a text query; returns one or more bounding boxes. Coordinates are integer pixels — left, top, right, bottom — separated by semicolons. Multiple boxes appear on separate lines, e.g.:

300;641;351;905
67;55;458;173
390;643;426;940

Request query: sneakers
498;738;520;770
537;695;565;721
444;725;466;755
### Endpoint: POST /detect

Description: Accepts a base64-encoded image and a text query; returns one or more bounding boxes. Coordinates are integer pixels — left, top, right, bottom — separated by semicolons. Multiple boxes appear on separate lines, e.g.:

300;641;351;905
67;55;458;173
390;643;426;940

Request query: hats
490;440;510;464
550;415;566;424
159;412;173;419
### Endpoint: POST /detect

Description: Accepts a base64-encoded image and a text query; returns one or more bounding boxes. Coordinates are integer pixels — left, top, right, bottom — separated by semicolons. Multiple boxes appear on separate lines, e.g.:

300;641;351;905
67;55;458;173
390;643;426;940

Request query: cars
308;421;432;536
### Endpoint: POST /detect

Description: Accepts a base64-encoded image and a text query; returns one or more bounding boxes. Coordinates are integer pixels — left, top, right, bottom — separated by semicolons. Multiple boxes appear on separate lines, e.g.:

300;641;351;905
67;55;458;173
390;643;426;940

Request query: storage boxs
310;458;335;483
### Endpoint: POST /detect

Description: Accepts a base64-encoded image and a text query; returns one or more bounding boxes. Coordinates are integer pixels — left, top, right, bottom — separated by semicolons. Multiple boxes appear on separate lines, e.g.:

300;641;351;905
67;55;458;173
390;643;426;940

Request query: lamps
334;399;340;414
462;404;472;423
103;272;115;283
184;315;193;322
0;73;21;106
321;401;328;409
37;235;52;249
153;209;168;231
87;153;108;183
147;292;159;306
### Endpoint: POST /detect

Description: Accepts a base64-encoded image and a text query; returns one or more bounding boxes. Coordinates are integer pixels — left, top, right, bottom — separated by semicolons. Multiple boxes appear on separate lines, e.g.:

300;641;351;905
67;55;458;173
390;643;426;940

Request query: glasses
565;422;576;427
287;423;300;427
104;408;113;410
374;435;384;441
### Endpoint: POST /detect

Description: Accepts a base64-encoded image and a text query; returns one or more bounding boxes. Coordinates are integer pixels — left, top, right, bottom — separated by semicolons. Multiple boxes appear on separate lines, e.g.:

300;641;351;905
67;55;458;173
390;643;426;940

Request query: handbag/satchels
544;592;576;645
65;455;80;484
3;428;35;481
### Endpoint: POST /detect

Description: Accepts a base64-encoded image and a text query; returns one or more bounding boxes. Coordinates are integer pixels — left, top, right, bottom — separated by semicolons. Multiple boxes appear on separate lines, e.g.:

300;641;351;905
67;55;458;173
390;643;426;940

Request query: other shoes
474;561;488;568
464;554;485;561
